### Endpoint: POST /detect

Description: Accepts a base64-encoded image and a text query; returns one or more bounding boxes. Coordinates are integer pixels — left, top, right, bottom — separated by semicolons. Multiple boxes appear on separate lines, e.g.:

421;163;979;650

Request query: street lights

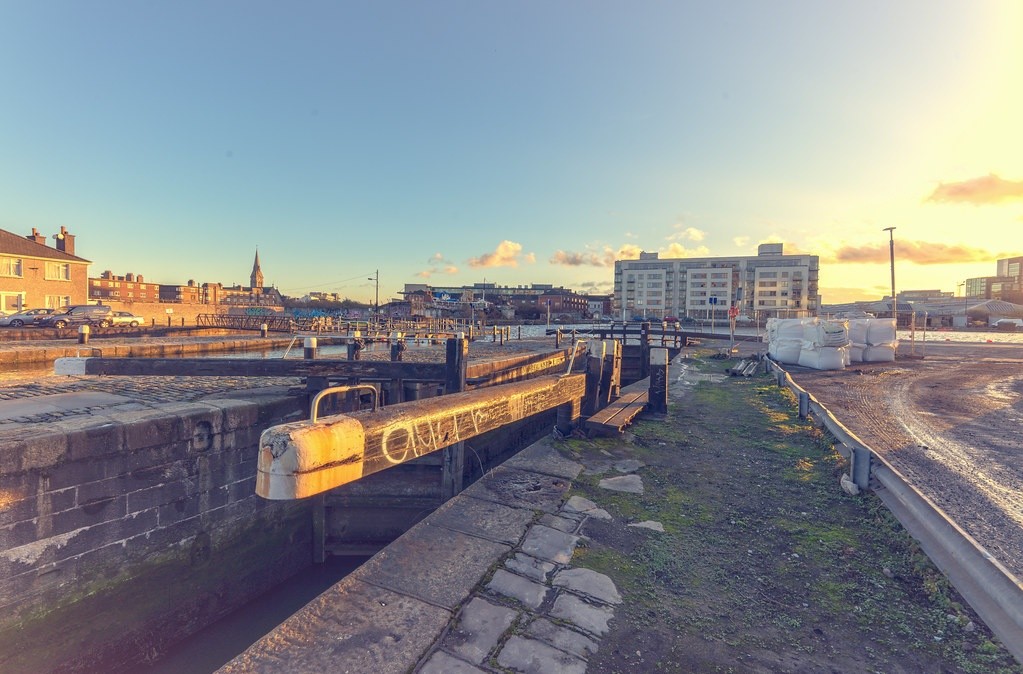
367;269;379;322
883;226;898;355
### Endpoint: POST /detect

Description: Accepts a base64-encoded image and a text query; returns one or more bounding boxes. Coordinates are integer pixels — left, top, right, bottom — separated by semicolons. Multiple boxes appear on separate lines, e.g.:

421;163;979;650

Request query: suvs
39;304;113;328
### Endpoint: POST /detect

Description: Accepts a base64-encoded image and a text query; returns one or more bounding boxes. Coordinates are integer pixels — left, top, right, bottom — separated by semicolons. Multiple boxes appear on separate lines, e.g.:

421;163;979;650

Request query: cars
0;308;55;328
631;315;698;324
112;310;145;328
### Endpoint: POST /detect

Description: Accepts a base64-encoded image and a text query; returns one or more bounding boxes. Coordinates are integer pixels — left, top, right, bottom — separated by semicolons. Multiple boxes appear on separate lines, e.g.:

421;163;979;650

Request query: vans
348;321;375;331
990;319;1023;327
